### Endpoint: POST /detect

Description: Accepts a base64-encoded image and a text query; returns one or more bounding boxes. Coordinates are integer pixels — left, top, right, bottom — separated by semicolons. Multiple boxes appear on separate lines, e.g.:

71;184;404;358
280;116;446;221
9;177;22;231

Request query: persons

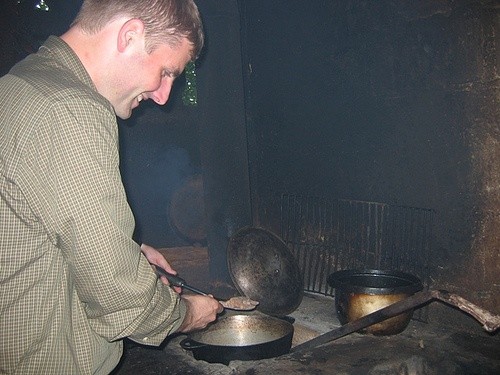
0;0;225;375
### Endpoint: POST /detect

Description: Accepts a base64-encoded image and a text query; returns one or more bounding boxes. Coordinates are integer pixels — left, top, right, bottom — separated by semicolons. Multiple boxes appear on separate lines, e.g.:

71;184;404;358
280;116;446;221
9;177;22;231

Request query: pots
180;315;295;366
328;268;424;335
